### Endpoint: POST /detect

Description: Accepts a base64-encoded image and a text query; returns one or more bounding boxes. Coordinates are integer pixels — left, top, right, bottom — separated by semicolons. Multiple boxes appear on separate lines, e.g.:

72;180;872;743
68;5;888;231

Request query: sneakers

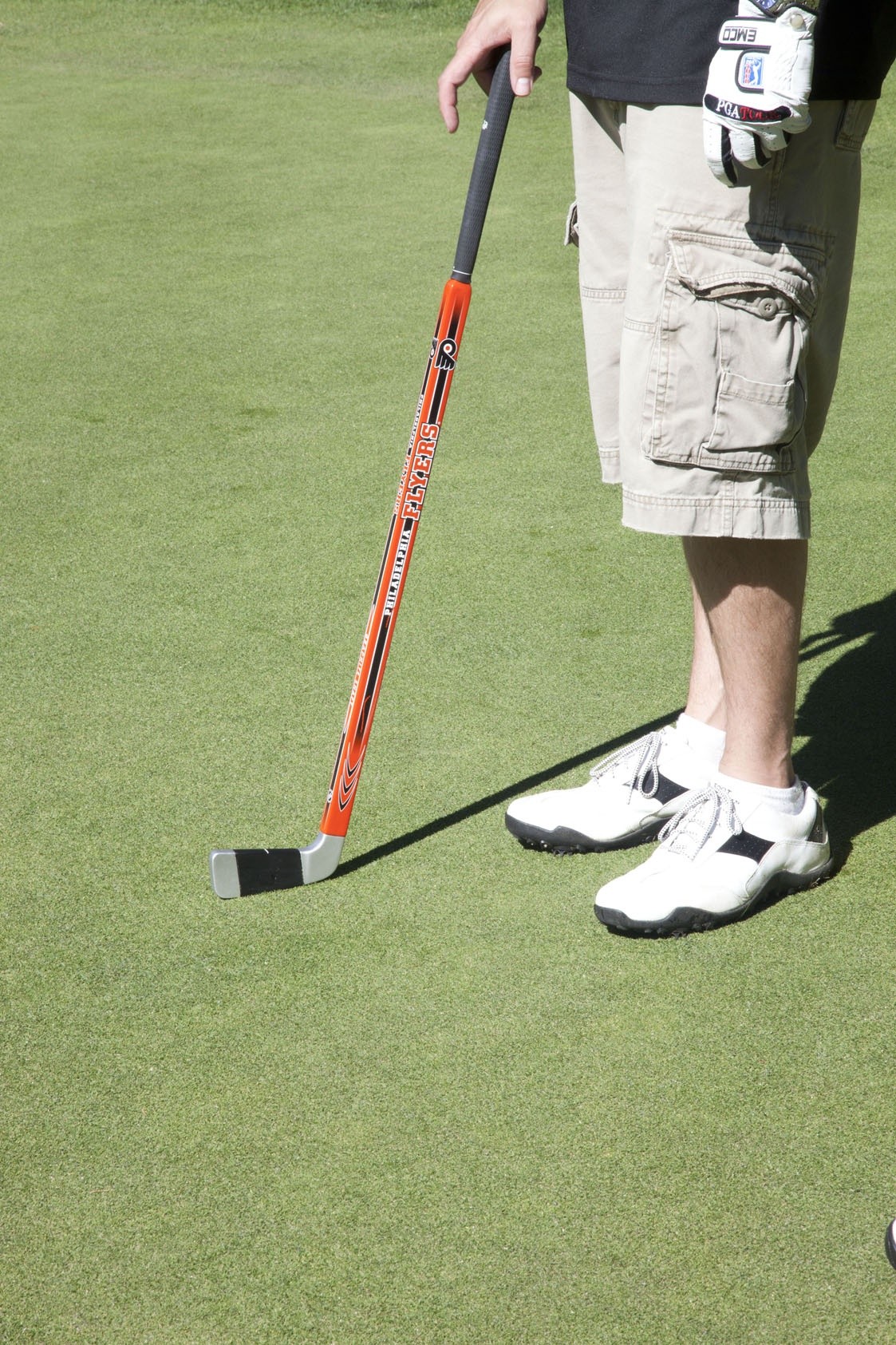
594;776;834;939
501;720;720;850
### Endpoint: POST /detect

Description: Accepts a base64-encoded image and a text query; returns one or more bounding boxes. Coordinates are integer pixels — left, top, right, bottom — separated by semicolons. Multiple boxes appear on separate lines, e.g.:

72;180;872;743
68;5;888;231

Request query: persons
437;0;896;937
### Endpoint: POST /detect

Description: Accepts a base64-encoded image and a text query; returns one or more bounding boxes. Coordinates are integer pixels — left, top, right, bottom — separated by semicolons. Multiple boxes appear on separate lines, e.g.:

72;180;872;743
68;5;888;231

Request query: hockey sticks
207;40;540;904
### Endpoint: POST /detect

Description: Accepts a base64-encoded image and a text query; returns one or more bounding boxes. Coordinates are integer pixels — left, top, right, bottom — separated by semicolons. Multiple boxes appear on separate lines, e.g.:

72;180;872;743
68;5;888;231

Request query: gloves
705;0;816;185
